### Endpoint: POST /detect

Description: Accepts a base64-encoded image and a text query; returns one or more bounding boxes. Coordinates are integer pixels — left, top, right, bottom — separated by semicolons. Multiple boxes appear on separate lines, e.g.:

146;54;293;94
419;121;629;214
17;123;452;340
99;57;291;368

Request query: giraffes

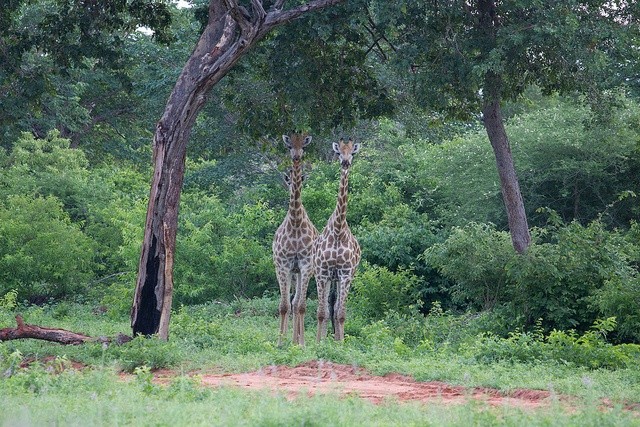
272;130;321;349
312;137;360;343
